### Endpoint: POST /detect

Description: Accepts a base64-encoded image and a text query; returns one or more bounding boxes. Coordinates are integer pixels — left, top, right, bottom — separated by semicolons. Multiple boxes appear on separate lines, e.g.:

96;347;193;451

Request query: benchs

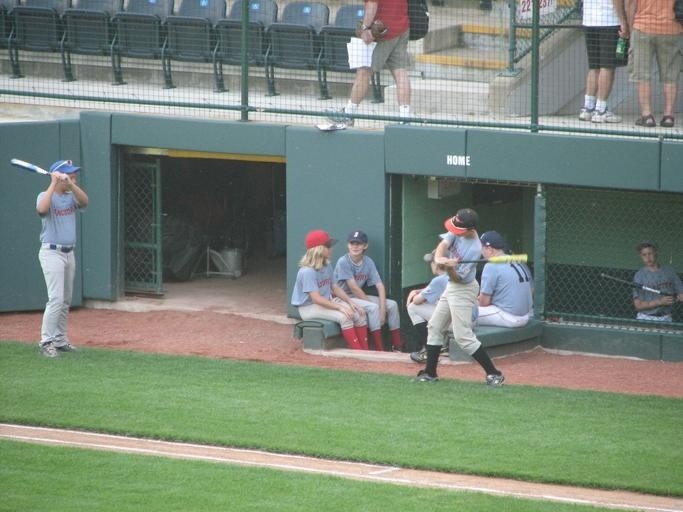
449;322;540;367
292;317;347;351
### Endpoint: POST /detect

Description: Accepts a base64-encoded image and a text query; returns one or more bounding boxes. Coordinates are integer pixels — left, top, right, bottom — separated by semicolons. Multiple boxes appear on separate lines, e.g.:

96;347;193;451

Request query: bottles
615;34;625;58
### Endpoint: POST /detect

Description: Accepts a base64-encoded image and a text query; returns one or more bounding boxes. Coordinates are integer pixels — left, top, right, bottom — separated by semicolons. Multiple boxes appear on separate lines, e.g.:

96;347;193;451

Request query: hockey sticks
601;272;678;297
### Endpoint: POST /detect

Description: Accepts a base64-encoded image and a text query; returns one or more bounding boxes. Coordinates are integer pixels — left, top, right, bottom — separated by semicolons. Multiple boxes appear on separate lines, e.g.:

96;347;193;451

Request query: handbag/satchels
407;0;430;40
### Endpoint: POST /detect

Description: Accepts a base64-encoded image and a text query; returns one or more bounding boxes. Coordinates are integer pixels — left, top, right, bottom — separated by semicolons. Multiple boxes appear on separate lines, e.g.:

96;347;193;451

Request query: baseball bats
11;159;68;181
445;254;531;263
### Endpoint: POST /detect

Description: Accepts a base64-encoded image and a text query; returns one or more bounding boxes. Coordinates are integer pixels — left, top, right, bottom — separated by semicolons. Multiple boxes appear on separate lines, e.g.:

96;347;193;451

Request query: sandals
659;115;674;127
635;114;656;127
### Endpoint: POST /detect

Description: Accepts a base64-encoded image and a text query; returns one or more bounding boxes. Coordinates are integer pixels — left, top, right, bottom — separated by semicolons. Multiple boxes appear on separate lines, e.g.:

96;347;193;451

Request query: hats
49;159;82;174
423;248;450;263
633;239;657;252
479;230;504;248
347;230;368;244
443;207;479;235
305;230;339;249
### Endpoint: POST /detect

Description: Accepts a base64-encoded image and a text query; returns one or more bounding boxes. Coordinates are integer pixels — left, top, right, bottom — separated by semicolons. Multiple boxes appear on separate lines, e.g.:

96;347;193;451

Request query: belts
50;244;72;252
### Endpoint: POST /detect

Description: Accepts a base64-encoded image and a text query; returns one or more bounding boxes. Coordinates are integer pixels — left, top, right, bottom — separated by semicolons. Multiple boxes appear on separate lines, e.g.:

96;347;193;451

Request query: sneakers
314;110;355;131
412;346;449;384
391;346;409;353
56;343;77;351
39;341;59;358
486;371;506;386
590;107;622;123
578;107;595;121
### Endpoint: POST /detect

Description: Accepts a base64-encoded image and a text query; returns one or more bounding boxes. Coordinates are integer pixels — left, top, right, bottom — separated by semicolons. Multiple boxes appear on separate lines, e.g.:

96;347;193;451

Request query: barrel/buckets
220;248;242;271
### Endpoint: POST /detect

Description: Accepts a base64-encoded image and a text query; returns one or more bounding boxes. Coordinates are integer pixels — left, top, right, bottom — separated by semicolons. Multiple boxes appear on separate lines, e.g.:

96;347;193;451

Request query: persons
626;0;683;127
36;160;89;358
316;0;411;131
413;208;506;388
333;230;408;353
290;229;369;350
476;231;533;329
579;0;629;124
632;240;683;322
406;249;479;364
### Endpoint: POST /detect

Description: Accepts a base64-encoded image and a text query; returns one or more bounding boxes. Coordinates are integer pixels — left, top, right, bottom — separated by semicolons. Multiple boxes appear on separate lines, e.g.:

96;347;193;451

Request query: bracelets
362;25;372;30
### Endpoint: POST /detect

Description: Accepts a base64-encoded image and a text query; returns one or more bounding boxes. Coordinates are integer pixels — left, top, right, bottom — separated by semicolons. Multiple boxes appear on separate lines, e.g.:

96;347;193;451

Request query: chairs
2;0;22;78
316;4;384;103
60;0;122;86
266;2;330;98
109;0;174;90
8;2;70;82
213;2;278;97
161;1;227;93
